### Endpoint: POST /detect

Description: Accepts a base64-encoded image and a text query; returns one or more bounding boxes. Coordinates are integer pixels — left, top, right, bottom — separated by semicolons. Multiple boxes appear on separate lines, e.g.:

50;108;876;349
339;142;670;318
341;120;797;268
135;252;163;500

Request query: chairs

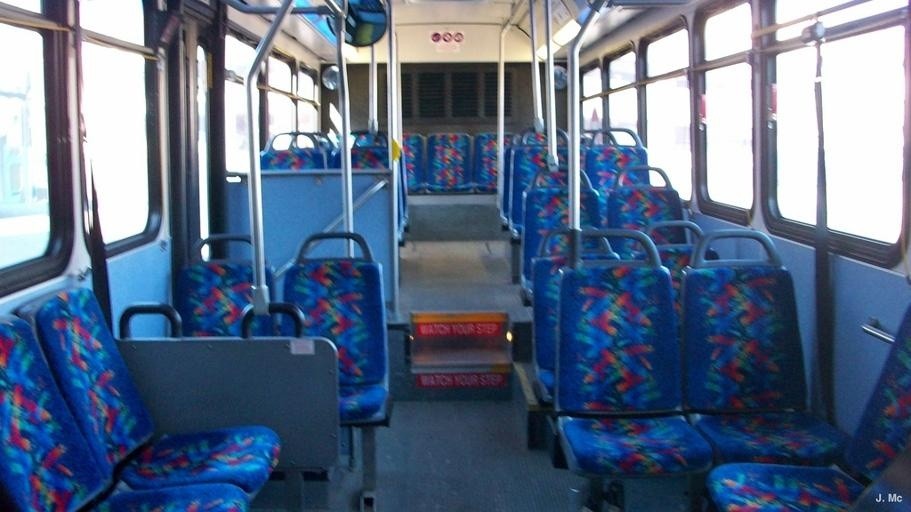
259;126;687;299
529;221;910;511
0;232;391;511
